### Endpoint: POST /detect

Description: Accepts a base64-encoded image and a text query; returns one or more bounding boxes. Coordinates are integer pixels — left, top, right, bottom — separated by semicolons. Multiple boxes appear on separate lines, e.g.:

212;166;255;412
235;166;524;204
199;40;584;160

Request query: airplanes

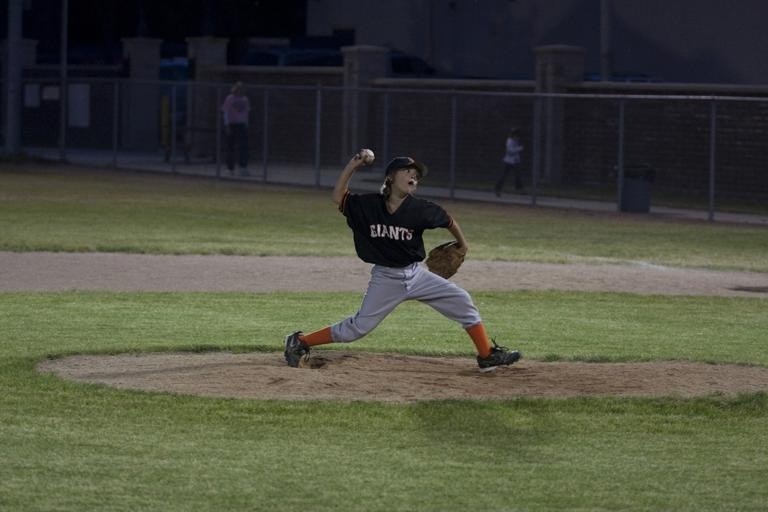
425;241;467;279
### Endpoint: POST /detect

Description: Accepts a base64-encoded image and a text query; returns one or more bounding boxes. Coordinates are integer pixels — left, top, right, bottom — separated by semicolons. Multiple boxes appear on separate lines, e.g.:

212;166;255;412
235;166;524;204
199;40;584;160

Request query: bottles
385;156;427;181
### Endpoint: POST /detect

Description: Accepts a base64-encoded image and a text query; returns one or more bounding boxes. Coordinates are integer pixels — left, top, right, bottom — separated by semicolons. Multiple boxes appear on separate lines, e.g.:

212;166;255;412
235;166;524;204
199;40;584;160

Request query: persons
283;145;522;369
220;81;251;173
494;127;525;199
163;71;190;165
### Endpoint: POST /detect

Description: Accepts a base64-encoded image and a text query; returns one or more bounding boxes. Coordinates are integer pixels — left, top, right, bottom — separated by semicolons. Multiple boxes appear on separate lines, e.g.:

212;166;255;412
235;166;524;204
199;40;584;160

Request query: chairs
477;348;520;374
285;331;310;367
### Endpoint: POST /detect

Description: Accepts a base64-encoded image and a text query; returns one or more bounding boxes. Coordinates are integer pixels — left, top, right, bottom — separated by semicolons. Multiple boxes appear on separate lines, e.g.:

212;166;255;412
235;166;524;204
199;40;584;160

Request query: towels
617;162;651;212
